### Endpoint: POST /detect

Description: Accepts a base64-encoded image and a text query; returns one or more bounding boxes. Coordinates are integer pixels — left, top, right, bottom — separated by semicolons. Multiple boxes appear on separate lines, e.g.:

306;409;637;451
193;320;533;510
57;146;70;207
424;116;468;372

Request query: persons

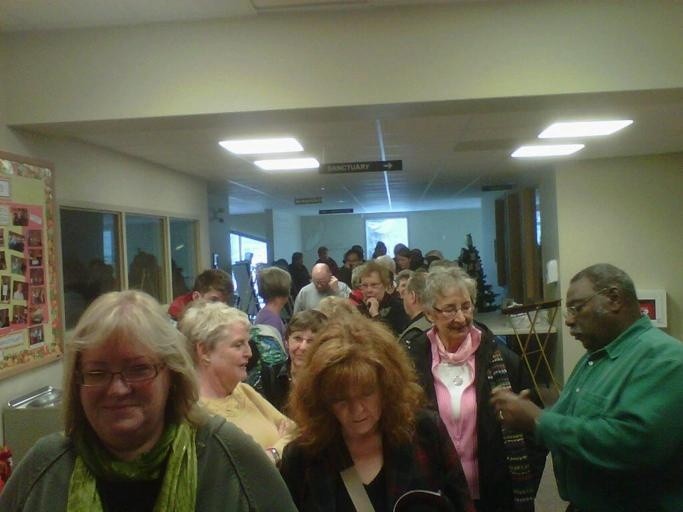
174;299;302;465
246;242;444;413
0;289;297;512
278;313;469;512
405;266;548;511
167;269;234;321
489;263;683;512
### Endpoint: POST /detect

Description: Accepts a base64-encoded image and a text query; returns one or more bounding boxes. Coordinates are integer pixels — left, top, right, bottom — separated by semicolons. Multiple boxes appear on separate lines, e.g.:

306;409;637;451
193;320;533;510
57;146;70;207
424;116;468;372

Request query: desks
475;306;557;390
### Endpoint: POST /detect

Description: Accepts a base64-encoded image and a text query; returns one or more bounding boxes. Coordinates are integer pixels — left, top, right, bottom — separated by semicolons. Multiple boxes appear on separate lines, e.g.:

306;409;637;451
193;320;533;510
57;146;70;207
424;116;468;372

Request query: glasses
433;302;474;318
562;288;608;321
78;363;165;388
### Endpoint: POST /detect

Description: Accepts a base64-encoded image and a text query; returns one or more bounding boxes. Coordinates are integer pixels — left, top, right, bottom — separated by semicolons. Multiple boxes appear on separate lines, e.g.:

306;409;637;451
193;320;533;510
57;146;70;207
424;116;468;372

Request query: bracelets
270;449;281;463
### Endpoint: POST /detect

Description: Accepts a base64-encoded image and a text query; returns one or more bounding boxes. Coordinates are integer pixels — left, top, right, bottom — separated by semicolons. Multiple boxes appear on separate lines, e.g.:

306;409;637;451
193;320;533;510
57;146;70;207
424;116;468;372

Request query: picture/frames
635;288;668;328
